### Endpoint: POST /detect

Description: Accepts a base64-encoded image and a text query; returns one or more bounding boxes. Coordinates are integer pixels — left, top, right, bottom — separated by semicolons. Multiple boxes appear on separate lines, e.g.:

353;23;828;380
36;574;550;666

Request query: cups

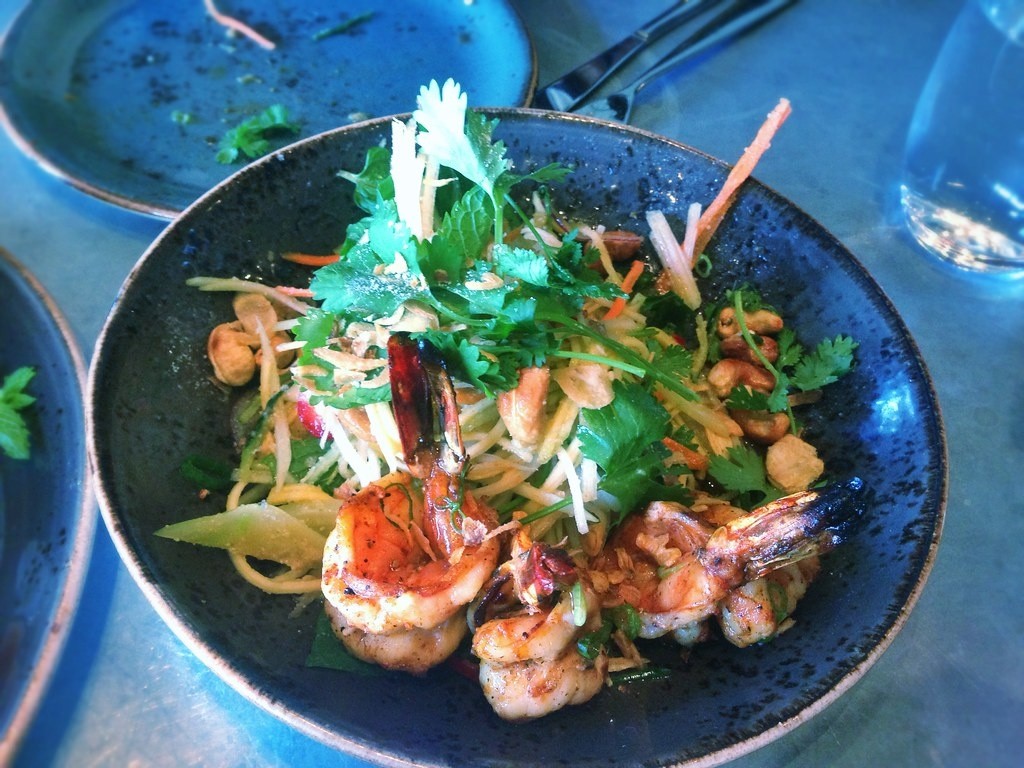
899;0;1024;282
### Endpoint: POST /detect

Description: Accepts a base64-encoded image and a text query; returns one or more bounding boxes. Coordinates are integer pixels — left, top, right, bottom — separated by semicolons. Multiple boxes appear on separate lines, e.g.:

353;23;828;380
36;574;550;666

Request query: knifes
529;0;717;112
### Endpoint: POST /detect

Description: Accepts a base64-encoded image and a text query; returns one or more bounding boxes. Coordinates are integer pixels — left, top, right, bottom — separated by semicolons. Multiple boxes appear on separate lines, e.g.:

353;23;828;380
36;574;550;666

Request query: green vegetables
292;75;860;680
212;104;299;165
0;365;42;459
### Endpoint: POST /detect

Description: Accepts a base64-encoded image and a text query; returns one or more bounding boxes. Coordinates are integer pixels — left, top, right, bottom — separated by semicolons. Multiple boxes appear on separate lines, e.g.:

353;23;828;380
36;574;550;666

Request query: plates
0;0;539;221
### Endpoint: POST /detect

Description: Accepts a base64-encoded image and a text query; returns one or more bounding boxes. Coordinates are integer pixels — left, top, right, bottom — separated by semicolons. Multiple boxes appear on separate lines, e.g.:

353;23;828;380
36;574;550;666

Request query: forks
571;0;802;124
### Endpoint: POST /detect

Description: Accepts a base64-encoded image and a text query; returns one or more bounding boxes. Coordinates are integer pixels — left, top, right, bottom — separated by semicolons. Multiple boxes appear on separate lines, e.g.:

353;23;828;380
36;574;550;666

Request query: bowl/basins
0;245;97;768
83;106;953;768
